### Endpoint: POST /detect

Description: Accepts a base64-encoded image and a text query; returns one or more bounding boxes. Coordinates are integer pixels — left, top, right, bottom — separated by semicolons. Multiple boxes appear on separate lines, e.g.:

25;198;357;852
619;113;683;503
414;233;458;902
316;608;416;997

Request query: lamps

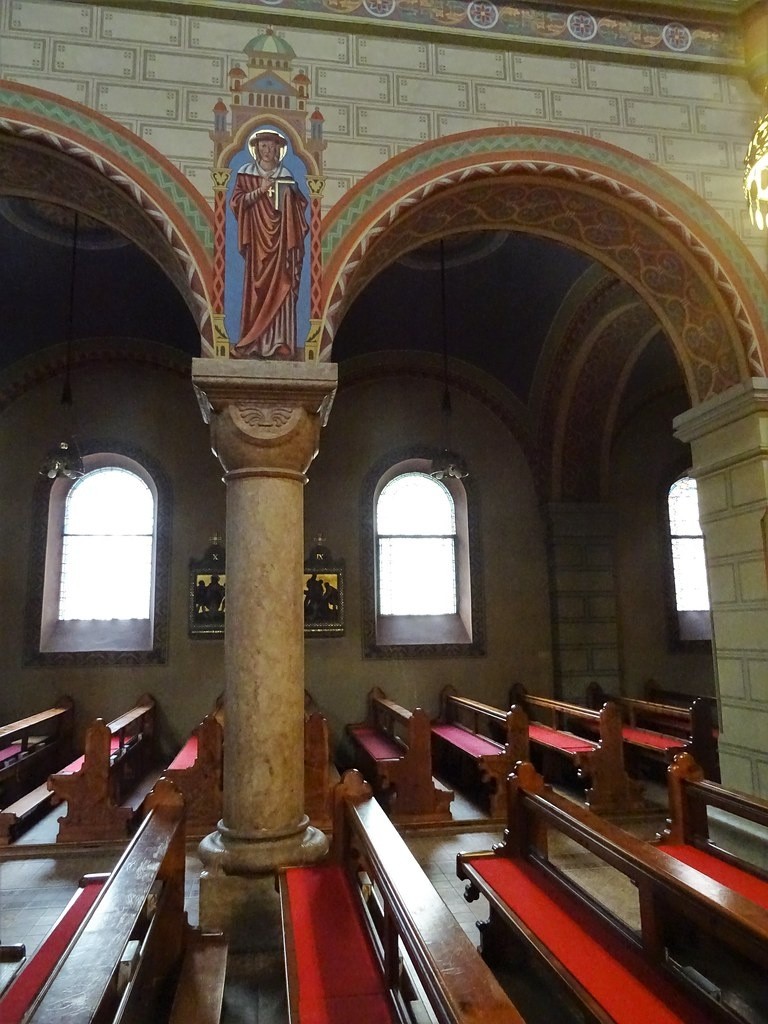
428;238;470;479
39;214;85;481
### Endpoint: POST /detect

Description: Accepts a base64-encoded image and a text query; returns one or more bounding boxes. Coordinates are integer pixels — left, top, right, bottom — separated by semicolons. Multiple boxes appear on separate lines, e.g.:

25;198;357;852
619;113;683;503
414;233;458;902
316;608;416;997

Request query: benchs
0;682;768;1024
